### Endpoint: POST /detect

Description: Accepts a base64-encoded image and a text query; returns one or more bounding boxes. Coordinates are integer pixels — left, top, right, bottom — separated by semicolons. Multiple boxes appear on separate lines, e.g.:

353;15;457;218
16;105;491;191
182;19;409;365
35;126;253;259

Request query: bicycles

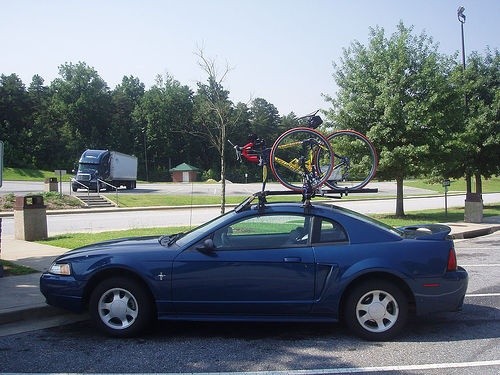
227;124;375;192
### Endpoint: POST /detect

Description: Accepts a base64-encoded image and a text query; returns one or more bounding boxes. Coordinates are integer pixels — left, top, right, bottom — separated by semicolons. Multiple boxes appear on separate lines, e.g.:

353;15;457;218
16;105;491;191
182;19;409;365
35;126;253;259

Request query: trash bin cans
464;193;484;223
44;177;58;193
13;194;48;241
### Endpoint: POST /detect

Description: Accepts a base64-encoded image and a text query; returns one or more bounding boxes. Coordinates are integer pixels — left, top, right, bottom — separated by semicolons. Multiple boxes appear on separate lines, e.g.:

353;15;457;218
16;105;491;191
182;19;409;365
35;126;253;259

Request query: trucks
71;149;138;192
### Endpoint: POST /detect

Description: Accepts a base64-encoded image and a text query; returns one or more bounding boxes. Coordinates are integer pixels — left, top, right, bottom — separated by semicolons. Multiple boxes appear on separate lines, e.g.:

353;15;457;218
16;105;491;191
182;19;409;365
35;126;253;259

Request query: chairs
281;227;305;245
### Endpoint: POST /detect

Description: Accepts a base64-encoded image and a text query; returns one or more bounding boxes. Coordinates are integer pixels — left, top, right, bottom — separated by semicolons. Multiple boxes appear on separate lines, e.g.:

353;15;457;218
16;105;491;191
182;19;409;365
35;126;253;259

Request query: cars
40;199;468;342
